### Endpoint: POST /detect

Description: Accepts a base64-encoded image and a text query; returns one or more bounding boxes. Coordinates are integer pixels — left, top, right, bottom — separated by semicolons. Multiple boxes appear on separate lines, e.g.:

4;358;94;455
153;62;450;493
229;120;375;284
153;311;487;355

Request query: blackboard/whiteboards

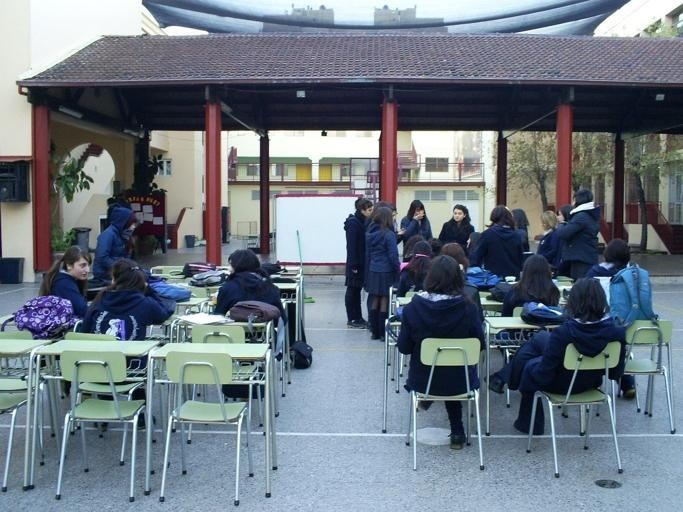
275;193;362;265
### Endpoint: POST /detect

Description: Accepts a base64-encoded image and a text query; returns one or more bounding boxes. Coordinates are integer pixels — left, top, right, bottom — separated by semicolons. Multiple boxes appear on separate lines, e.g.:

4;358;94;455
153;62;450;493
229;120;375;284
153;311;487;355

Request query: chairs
159;350;254;506
611;319;675;434
0;330;55;466
0;392;40;493
503;306;525;409
64;331;156;465
525;340;623;479
55;349;155;503
405;337;485;472
190;324;263;427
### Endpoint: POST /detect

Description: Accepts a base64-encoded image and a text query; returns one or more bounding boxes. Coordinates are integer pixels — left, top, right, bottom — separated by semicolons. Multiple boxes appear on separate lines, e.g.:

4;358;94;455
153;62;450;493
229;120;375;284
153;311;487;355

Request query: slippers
370;320;377;339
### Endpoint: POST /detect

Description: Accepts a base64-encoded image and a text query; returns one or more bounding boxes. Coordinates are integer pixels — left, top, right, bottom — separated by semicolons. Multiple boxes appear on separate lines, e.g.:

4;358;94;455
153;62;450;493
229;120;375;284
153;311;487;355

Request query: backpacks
609;266;660;330
182;258;216;279
188;268;231;288
0;296;81;344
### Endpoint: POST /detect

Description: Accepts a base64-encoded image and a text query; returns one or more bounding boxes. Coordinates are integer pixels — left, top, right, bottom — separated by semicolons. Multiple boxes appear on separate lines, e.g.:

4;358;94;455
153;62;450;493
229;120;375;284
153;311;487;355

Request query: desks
0;339;52;491
395;294;413;393
273;281;302;343
479;297;504;313
176;319;281;419
144;341;278;498
29;339;160;493
162;314;181;342
484;315;561;435
175;295;209;315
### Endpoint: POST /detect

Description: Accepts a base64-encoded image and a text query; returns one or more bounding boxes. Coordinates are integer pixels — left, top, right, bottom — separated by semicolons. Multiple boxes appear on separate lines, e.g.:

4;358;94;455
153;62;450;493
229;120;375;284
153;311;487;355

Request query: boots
379;320;385;341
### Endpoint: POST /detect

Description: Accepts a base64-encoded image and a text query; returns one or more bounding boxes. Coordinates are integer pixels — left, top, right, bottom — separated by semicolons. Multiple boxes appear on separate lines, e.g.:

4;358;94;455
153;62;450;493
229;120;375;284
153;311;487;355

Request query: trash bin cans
185;234;196;248
0;257;25;284
71;226;92;256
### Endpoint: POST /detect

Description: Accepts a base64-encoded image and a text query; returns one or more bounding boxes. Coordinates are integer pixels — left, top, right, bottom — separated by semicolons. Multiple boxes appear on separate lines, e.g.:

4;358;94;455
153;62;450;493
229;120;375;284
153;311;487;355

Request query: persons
438;204;475;255
467;206;524;281
555;189;602;282
585;238;631;279
544;205;573;277
91;208;138;287
397;254;486;450
363;201;402;338
396;241;434;297
214;249;288;330
536;211;559;255
79;258;169;430
37;246;94;316
510;208;531;254
400;200;432;263
344;198;374;333
501;254;560;319
484;278;627;435
439;242;484;324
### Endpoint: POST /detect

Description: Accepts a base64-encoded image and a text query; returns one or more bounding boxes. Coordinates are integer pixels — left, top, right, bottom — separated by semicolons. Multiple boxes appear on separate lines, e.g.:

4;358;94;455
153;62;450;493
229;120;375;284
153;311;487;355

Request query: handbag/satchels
229;300;281;337
292;340;314;369
520;302;569;325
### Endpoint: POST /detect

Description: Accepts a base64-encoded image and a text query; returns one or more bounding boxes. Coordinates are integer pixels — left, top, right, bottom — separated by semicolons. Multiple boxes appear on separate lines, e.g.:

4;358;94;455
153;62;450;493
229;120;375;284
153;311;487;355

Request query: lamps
122;128;139;137
138;123;145;138
655;93;665;101
58;104;83;119
295;89;307;98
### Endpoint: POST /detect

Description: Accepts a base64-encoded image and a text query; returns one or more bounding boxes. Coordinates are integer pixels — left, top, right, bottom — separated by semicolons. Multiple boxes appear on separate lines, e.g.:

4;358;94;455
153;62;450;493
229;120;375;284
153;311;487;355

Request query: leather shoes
347;319;364;329
360;318;368;325
484;373;504;393
137;415;155;429
450;434;466;450
418;400;432;410
623;384;635;397
